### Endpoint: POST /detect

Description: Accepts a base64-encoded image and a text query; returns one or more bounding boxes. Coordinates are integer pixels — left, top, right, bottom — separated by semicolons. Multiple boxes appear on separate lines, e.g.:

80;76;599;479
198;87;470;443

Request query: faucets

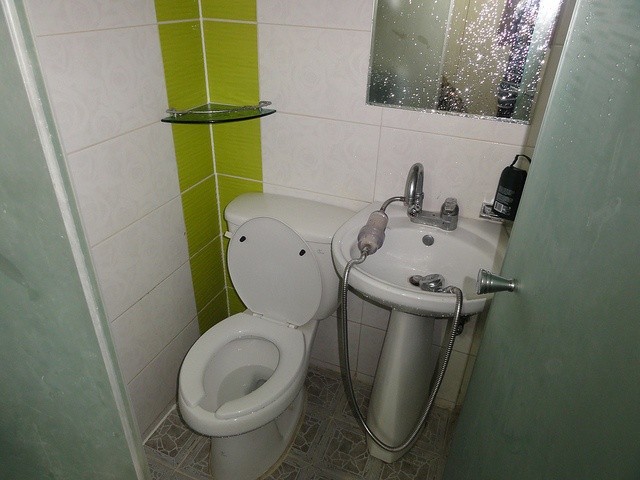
403;163;425;218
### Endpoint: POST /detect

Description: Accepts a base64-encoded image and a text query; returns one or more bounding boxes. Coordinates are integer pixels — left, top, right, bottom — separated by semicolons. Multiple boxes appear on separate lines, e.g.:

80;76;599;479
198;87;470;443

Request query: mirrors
365;1;570;127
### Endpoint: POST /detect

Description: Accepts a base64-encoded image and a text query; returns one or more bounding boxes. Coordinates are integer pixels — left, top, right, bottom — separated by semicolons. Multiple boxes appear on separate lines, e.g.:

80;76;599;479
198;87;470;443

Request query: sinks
331;199;510;319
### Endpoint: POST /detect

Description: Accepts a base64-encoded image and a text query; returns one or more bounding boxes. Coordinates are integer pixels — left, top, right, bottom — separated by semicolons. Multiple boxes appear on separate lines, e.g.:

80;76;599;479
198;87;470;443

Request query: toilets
177;193;360;480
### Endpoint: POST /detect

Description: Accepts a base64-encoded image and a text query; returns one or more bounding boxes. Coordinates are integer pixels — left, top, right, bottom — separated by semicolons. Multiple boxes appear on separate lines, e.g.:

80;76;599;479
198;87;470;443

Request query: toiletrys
492;154;531;221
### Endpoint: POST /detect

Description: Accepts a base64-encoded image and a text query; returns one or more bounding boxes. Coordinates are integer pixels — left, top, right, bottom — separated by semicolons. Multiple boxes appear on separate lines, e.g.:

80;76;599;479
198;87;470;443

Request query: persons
439;72;473;113
